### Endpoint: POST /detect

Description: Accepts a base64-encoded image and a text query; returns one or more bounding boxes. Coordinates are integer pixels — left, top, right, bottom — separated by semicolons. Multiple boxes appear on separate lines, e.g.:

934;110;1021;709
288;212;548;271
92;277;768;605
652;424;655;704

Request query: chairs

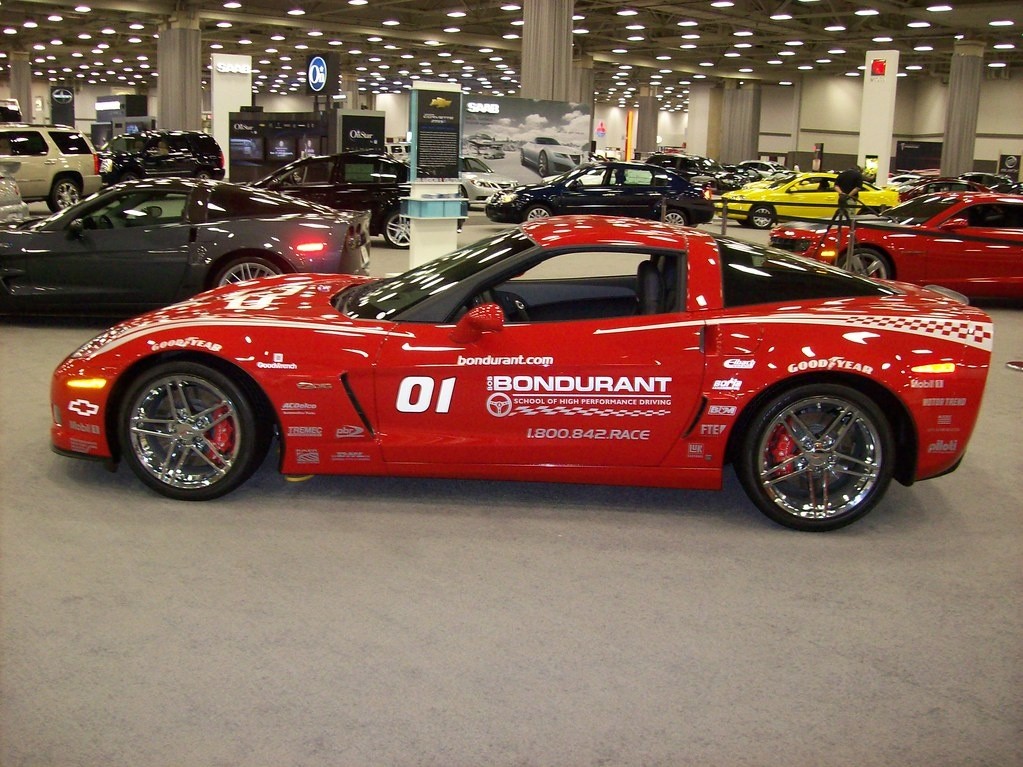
631;261;664;315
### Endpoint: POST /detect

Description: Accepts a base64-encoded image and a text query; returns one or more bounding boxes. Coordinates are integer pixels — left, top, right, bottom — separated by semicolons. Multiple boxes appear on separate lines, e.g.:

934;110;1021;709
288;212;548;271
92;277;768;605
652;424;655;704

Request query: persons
834;166;862;212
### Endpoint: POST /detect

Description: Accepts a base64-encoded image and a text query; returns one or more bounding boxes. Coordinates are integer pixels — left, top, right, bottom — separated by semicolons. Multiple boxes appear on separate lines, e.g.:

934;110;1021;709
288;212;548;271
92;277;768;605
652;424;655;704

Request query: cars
991;181;1023;195
249;151;470;249
458;156;519;208
713;171;901;229
770;191;1023;298
888;174;922;186
0;167;30;220
742;170;835;191
0;176;373;316
520;137;589;177
645;153;750;195
956;172;1013;191
733;161;792;178
98;129;225;191
0;122;102;213
899;177;997;203
485;161;715;229
483;145;505;159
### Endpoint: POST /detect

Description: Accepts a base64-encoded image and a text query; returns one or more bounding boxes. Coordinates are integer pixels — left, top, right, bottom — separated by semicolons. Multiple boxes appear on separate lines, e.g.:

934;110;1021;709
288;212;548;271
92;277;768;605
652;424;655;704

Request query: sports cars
48;217;992;532
